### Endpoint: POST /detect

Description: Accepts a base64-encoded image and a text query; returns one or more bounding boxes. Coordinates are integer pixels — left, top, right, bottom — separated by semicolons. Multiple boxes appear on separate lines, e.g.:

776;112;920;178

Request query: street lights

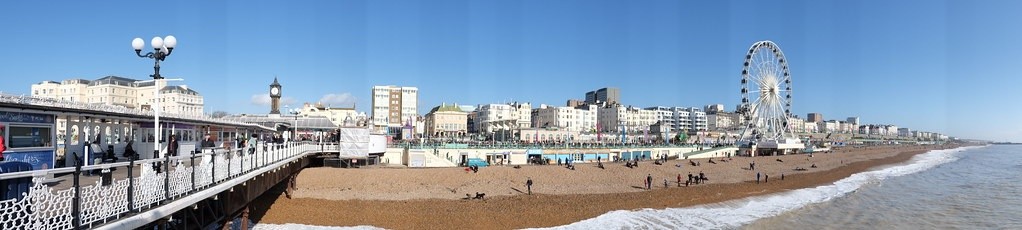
133;34;176;174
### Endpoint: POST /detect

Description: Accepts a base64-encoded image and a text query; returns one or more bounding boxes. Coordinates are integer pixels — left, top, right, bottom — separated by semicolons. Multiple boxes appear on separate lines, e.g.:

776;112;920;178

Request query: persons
0;125;7;173
108;148;119;160
812;163;816;167
391;137;784;170
240;134;258;155
664;179;668;187
125;140;137;159
644;174;652;189
757;172;760;182
170;135;178;162
316;132;340;145
299;135;306;140
202;134;215;154
765;173;768;182
525;177;532;194
271;135;284;149
678;171;705;187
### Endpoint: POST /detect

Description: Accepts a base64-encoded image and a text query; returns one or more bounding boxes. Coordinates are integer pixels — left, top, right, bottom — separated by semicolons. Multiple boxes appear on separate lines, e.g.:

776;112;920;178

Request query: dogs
476;192;485;198
